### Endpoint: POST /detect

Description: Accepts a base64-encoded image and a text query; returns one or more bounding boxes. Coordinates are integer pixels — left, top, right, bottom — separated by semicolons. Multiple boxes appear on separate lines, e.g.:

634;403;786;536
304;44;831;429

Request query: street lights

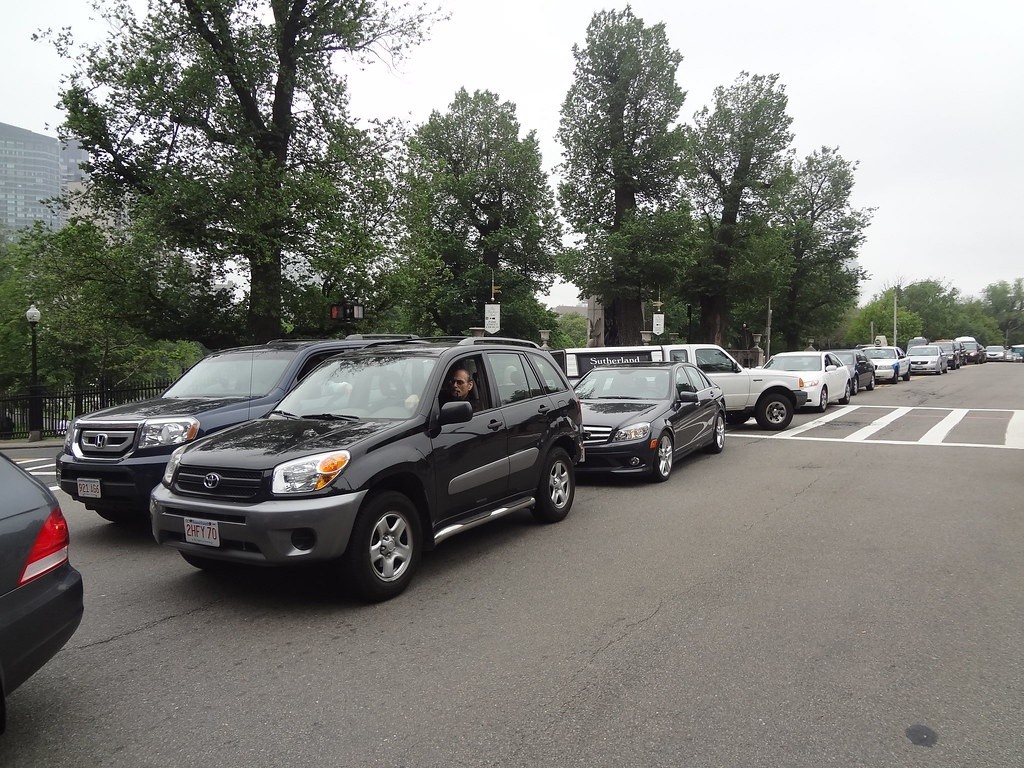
26;305;40;386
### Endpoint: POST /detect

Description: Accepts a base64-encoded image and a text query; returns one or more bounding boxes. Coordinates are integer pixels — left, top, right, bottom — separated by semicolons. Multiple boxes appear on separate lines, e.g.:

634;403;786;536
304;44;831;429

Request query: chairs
369;370;407;413
610;377;633;395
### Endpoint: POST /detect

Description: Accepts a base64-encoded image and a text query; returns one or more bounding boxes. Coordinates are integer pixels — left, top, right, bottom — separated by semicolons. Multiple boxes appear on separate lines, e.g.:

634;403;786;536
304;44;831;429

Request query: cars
822;350;876;396
762;351;851;414
573;361;727;483
0;452;85;737
861;346;912;385
905;345;949;375
928;336;1024;370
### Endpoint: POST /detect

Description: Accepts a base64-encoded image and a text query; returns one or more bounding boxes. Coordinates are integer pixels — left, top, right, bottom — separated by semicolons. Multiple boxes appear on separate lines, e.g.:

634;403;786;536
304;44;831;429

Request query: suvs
56;333;431;526
148;336;585;604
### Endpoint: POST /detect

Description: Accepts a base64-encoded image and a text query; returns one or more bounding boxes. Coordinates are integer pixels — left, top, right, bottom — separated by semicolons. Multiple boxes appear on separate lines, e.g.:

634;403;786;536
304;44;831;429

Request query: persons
654;373;668;394
60;415;71;434
404;369;482;414
310;373;354;397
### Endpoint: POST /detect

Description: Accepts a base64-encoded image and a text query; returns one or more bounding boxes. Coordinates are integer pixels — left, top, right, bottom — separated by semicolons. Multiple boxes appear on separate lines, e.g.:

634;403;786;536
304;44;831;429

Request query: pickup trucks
552;344;807;430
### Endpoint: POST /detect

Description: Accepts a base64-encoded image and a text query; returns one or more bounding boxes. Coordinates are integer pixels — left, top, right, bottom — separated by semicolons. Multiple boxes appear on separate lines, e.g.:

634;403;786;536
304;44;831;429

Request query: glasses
448;379;470;385
